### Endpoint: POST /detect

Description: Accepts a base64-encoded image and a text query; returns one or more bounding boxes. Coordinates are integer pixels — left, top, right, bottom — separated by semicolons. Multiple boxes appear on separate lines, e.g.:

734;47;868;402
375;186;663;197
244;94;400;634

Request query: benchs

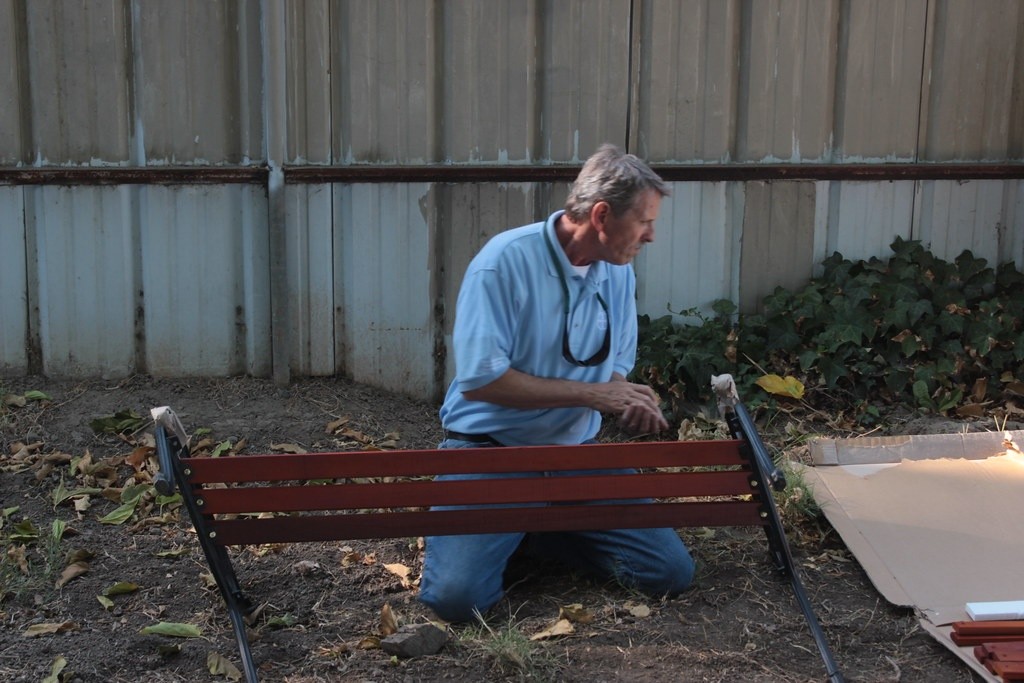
153;373;846;683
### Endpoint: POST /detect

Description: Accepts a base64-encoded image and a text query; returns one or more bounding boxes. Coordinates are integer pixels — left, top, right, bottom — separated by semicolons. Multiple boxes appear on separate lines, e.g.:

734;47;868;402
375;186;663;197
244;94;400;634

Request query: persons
421;143;695;622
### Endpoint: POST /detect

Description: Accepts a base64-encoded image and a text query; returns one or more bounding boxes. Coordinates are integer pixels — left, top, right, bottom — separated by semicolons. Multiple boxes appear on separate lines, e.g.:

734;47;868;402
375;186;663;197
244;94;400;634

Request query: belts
443;423;501;449
